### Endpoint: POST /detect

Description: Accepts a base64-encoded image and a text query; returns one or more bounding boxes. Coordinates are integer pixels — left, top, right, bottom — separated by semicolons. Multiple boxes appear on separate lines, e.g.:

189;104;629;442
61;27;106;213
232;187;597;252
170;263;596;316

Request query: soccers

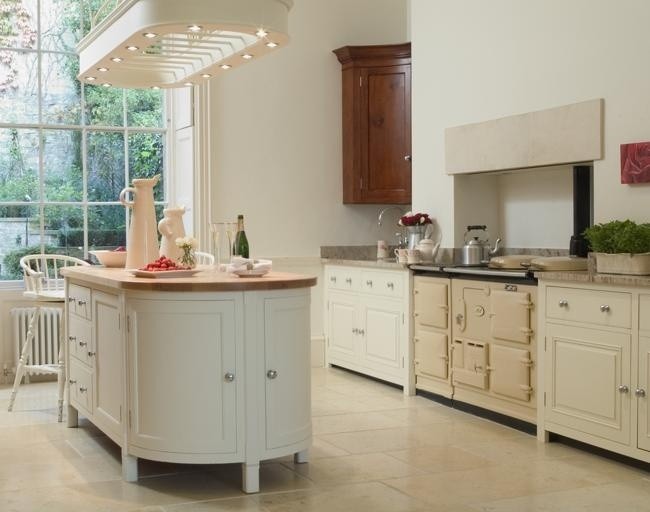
116;246;126;251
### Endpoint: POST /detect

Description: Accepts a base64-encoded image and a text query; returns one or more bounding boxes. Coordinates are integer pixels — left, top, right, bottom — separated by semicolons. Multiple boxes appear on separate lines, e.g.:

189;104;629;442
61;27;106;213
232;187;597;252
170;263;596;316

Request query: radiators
13;308;63;383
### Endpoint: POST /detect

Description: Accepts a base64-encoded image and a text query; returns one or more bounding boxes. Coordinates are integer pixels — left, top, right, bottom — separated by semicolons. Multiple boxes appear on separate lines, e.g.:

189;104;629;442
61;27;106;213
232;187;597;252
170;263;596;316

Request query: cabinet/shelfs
340;57;409;203
410;275;538;426
60;266;312;493
213;214;249;271
538;280;650;466
323;265;413;394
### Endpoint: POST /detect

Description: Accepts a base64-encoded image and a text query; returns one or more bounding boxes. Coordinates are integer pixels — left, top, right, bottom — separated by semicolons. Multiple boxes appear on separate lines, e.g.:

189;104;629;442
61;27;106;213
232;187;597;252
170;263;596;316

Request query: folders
13;308;63;383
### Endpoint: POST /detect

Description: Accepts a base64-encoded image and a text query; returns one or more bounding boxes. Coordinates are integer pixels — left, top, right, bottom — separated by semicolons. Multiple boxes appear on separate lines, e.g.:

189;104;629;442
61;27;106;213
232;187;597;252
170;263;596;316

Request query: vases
177;236;199;267
406;225;427;249
177;253;192;267
398;212;432;226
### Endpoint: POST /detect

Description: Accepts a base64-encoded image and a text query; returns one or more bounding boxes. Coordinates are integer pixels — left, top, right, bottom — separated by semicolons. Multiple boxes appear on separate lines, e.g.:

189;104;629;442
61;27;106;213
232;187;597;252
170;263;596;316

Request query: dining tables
581;219;650;275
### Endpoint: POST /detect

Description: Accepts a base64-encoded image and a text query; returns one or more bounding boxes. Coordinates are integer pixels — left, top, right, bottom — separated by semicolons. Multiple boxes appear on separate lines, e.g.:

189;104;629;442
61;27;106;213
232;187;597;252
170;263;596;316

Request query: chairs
9;251;81;425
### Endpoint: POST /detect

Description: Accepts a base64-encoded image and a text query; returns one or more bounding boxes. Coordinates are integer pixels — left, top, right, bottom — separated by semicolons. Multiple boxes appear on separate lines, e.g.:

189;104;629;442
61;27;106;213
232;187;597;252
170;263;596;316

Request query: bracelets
88;249;128;268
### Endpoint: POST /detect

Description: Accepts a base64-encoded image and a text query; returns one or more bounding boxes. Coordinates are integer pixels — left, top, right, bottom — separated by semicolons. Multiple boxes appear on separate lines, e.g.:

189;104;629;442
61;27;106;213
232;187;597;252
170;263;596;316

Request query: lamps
9;251;81;425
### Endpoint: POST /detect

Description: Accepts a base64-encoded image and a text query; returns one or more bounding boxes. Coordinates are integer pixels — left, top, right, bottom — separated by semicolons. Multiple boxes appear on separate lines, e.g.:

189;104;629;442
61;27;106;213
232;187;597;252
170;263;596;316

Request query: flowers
398;212;432;226
177;236;199;267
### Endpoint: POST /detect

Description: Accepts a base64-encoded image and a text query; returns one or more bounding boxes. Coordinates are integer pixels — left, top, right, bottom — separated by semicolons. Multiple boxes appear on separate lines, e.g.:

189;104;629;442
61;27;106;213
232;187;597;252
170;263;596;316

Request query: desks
24;194;31;247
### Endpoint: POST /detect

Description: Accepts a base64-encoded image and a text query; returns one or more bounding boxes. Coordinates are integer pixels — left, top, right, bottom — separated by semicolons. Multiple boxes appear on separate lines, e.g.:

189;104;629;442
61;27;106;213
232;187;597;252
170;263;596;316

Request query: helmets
120;268;201;280
231;270;267;277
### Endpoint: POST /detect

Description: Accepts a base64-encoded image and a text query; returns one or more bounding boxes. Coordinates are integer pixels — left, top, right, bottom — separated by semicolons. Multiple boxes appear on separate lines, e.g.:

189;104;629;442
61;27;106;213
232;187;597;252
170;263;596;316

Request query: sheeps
377;207;407;245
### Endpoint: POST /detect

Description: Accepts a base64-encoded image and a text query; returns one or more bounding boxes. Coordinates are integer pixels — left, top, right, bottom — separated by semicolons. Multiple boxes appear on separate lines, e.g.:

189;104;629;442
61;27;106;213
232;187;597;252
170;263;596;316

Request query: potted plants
581;219;650;275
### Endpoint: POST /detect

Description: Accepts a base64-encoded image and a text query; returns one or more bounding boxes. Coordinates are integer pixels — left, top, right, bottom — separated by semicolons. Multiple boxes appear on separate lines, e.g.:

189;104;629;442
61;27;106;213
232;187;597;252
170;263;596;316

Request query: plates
340;57;409;203
410;275;538;426
538;280;650;466
323;265;413;394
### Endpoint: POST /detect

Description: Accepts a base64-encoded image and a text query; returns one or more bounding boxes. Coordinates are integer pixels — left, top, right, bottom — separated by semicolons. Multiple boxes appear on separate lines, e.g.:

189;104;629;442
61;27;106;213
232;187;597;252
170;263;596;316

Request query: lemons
461;225;501;268
119;175;190;275
415;238;441;266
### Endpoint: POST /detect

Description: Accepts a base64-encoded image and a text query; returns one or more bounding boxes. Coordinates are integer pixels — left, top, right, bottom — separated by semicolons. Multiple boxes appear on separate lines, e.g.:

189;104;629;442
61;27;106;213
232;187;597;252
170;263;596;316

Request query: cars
376;241;392;259
393;248;420;265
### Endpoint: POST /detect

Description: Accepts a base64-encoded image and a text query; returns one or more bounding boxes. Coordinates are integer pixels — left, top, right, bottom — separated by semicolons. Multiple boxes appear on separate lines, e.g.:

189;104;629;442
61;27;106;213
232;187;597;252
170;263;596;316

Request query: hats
57;215;124;245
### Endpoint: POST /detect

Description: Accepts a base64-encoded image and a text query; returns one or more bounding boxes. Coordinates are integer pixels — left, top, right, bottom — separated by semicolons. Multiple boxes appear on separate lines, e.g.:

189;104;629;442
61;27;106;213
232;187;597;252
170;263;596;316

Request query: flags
177;253;192;267
406;225;427;249
